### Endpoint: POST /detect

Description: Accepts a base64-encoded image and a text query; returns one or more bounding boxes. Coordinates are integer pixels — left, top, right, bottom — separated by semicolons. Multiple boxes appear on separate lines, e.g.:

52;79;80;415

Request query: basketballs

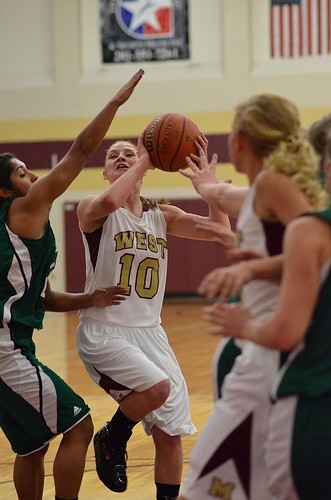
143;112;202;173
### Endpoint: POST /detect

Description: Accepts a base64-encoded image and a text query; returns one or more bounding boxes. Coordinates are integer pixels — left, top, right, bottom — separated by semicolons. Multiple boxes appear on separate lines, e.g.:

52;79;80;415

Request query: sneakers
94;422;129;493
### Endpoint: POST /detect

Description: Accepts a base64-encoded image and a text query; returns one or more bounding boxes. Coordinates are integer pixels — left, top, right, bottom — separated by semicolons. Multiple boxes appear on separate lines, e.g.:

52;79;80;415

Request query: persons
0;69;145;500
204;113;330;500
180;94;328;500
76;129;232;500
